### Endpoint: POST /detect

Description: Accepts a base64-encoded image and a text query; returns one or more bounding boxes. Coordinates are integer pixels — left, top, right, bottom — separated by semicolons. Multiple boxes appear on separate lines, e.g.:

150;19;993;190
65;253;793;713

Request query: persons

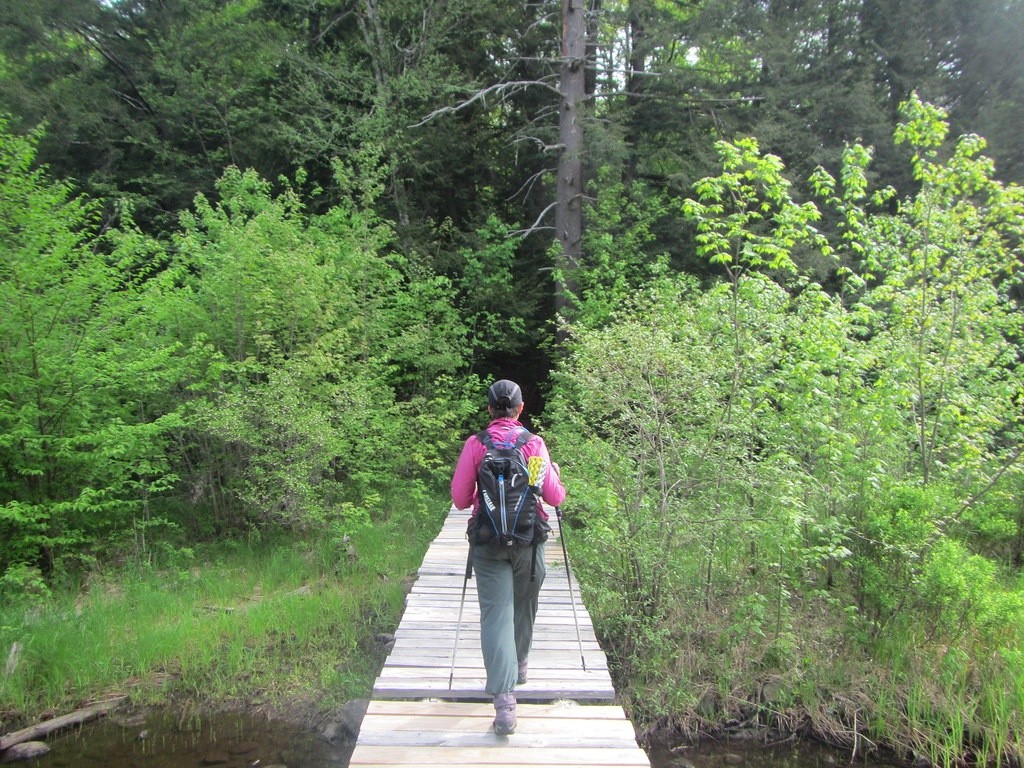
450;380;566;735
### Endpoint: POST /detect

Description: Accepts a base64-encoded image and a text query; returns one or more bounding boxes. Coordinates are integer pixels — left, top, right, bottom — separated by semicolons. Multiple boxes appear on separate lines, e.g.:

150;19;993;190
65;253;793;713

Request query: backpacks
466;429;552;582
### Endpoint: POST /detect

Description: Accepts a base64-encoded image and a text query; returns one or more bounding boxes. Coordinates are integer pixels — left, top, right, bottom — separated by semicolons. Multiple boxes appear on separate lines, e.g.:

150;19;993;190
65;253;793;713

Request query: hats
488;380;522;410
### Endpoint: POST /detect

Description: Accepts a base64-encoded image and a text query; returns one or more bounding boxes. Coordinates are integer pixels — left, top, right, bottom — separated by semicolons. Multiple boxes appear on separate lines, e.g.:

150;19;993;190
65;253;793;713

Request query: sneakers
493;693;517;735
517;658;528;683
